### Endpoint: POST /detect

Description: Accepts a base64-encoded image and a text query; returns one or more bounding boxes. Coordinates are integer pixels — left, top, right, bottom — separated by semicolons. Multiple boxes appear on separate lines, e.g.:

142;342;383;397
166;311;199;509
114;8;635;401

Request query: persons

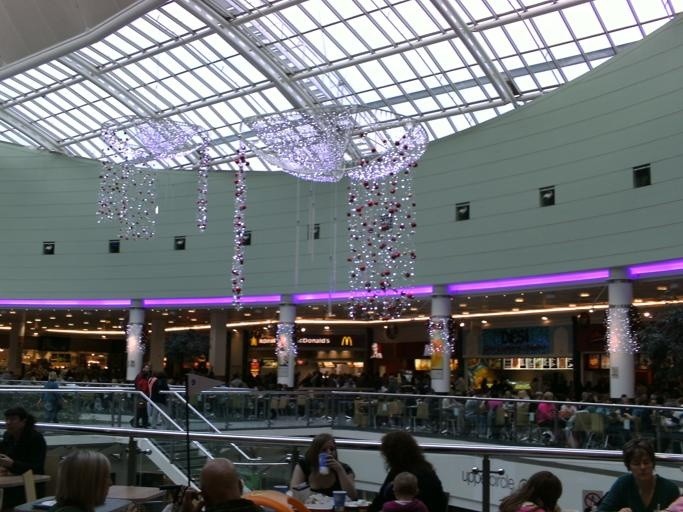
499;471;562;512
367;431;448;512
380;472;429;512
0;364;683;454
370;342;383;359
0;407;46;512
289;434;358;500
49;451;113;512
179;458;277;512
596;438;680;512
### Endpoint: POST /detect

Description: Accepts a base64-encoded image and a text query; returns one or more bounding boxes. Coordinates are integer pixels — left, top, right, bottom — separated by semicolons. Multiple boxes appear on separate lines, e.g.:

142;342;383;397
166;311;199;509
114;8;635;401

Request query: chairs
234;387;682;448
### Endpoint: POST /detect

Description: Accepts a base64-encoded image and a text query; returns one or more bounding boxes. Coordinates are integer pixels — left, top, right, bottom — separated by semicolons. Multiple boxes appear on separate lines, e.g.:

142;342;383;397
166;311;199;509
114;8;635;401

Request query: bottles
519;357;565;368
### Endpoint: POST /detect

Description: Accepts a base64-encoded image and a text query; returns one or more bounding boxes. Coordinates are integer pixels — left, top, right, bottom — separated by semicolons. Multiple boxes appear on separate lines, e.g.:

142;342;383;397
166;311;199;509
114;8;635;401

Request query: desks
1;472;170;512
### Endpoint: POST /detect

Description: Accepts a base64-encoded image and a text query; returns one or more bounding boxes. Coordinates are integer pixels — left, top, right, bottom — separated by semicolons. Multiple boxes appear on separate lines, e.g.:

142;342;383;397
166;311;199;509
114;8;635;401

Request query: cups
273;485;287;495
318;452;329;475
332;490;347;512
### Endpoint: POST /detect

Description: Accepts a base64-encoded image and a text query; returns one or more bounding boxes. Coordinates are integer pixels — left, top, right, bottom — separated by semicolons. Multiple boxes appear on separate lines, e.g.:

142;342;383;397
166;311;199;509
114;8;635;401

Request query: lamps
230;82;428;327
93;87;210;242
